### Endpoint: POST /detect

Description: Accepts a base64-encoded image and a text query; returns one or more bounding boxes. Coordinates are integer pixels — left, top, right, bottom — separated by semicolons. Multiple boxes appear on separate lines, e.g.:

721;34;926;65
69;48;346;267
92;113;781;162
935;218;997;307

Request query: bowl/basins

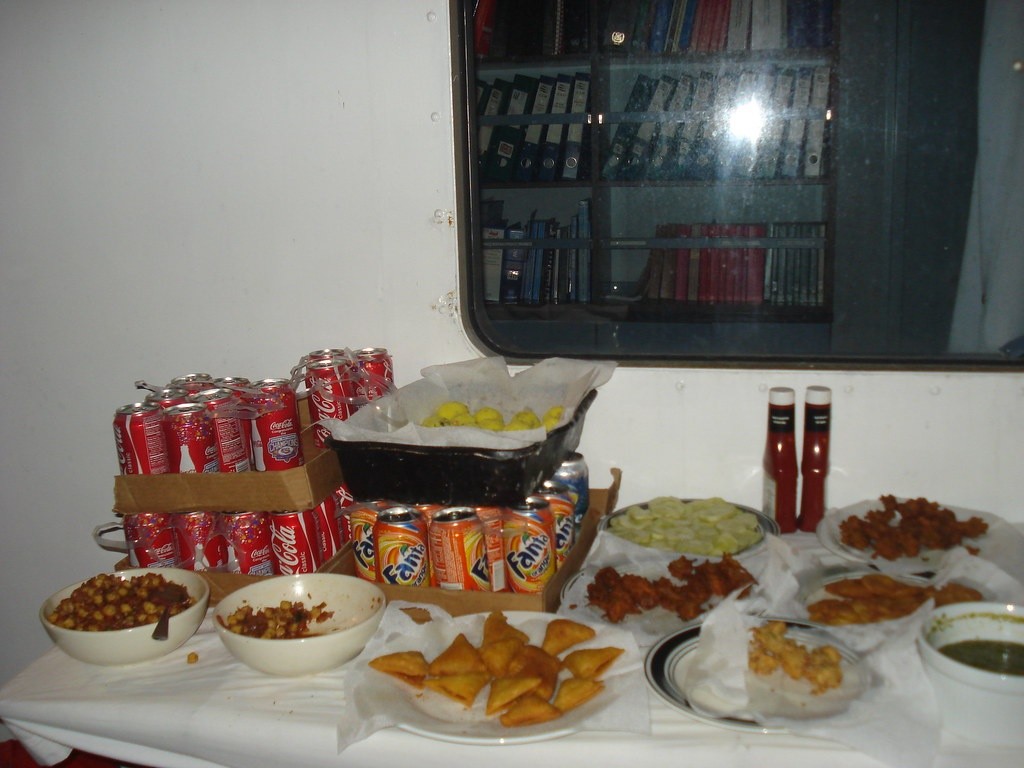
37;567;210;665
212;572;386;677
914;601;1024;742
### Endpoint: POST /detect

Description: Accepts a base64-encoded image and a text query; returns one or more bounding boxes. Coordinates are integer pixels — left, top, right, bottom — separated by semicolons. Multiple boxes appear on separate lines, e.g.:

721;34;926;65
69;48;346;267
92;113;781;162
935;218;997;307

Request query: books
473;0;589;61
604;223;824;308
481;198;590;301
602;0;824;55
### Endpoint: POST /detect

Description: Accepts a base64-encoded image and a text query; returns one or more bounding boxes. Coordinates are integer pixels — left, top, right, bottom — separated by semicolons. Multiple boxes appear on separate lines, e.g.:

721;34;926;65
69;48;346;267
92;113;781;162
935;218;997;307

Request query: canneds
111;373;305;473
121;480;352;576
304;348;393;448
345;452;588;594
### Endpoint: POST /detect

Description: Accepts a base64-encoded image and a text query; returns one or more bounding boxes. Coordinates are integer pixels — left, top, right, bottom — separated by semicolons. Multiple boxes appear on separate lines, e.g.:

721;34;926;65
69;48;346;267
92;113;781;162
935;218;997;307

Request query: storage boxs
115;375;622;623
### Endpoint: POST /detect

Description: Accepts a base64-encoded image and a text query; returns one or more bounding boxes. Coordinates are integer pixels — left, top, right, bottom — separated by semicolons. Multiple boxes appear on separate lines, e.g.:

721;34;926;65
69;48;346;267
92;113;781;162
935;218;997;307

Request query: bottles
760;387;798;534
799;386;832;532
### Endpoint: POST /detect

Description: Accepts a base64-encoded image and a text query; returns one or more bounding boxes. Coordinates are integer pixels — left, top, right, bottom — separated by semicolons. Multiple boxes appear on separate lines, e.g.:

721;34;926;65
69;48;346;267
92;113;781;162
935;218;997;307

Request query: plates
813;497;1009;574
561;563;757;644
364;606;624;743
643;620;873;735
597;497;781;556
788;572;931;634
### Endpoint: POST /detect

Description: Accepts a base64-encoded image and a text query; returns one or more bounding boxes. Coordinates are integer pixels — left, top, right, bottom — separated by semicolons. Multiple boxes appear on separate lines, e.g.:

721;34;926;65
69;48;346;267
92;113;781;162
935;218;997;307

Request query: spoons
148;583;188;641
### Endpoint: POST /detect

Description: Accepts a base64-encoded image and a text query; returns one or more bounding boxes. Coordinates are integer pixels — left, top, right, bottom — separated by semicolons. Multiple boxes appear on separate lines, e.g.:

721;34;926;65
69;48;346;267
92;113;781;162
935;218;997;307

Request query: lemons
419;402;566;435
604;495;762;556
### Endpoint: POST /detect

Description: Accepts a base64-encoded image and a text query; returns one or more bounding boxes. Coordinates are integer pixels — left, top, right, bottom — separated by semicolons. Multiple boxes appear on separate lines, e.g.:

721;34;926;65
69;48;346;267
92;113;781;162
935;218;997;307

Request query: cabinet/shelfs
461;0;835;321
